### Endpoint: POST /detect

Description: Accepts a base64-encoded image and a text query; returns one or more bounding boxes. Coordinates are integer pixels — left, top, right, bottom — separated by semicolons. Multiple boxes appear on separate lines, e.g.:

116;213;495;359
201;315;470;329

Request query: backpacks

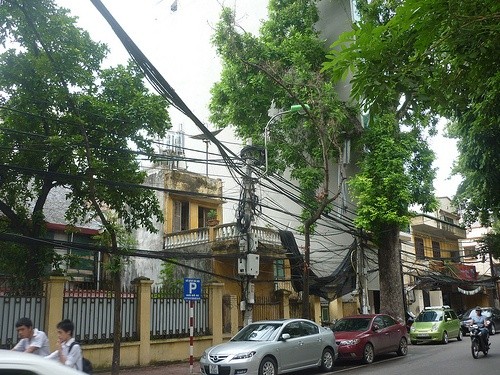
69;341;92;375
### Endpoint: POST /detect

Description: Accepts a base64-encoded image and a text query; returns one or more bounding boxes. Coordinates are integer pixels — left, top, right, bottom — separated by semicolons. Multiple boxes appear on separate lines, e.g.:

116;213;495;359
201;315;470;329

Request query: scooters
467;324;491;359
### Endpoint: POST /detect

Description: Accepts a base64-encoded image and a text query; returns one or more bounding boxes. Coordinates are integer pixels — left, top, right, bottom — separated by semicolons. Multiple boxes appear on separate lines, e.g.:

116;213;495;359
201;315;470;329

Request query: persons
11;318;49;358
44;318;83;372
470;308;488;342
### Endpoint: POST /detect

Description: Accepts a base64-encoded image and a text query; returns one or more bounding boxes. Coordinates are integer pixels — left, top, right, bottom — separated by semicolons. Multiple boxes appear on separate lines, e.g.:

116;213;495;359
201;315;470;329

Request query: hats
476;306;481;310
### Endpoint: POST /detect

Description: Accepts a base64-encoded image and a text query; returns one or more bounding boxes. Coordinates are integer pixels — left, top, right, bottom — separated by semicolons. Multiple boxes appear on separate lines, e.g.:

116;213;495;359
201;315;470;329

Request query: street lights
257;104;310;179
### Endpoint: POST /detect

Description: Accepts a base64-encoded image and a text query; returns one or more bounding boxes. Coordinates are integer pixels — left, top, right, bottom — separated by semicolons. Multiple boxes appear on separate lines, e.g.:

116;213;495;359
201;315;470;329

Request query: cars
0;348;89;375
332;313;408;363
199;319;340;375
406;311;416;330
409;305;462;346
460;306;499;336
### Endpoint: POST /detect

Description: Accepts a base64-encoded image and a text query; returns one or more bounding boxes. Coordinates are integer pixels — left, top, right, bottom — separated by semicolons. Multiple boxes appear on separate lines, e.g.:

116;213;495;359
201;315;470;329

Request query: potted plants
207;209;219;225
